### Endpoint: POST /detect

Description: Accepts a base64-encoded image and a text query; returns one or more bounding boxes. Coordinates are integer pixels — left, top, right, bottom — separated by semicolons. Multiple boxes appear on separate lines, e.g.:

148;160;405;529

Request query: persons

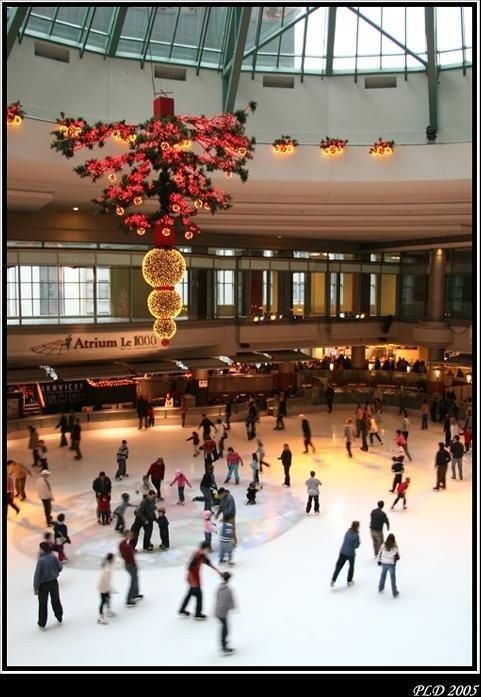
120;529;144;604
53;513;72;563
371;500;391;557
219;513;236;565
96;552;116;624
217;572;236;653
179;541;224;618
34;541;64;627
331;520;361;586
4;352;472;552
377;532;401;597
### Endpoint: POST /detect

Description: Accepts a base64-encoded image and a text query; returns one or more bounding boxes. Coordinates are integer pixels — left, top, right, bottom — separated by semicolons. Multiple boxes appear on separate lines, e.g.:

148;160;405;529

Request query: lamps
141;244;185;348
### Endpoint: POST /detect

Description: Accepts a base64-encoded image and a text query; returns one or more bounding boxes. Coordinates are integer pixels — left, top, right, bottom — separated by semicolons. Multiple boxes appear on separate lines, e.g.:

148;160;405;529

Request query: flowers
7;99;26;130
49;98;258;246
272;134;397;157
